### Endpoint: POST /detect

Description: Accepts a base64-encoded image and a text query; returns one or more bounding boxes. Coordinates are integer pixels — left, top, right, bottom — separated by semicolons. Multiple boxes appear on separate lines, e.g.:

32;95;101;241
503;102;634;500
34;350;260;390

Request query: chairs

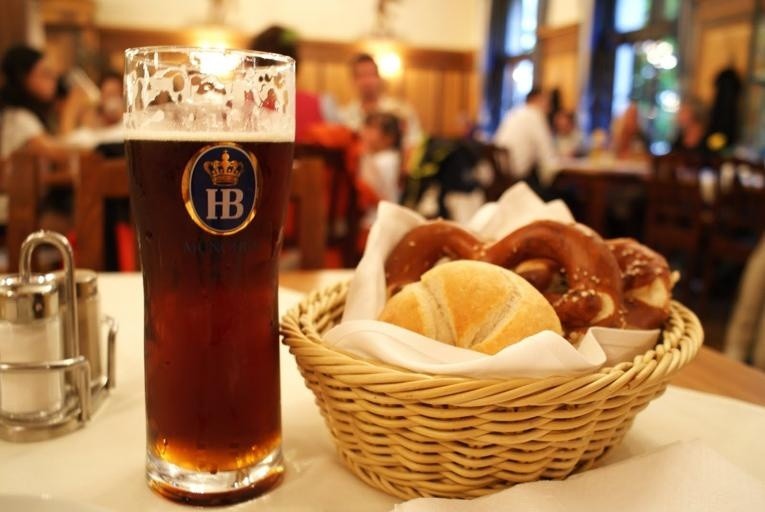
72;147;333;271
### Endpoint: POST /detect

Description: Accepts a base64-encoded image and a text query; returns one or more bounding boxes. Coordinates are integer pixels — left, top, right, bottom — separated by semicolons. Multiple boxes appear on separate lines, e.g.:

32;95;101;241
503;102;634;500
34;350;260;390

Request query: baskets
275;275;705;502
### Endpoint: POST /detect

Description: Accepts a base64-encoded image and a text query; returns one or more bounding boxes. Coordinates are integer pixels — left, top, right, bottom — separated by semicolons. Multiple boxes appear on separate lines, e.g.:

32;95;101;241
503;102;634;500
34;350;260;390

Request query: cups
123;44;296;505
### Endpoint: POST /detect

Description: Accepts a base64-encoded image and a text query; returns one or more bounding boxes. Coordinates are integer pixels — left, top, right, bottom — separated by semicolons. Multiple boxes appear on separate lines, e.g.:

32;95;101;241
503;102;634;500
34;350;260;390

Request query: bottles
1;272;65;420
47;270;105;382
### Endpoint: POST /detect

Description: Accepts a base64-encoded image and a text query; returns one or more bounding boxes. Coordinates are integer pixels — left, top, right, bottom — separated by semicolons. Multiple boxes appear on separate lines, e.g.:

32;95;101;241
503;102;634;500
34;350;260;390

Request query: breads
379;219;672;355
